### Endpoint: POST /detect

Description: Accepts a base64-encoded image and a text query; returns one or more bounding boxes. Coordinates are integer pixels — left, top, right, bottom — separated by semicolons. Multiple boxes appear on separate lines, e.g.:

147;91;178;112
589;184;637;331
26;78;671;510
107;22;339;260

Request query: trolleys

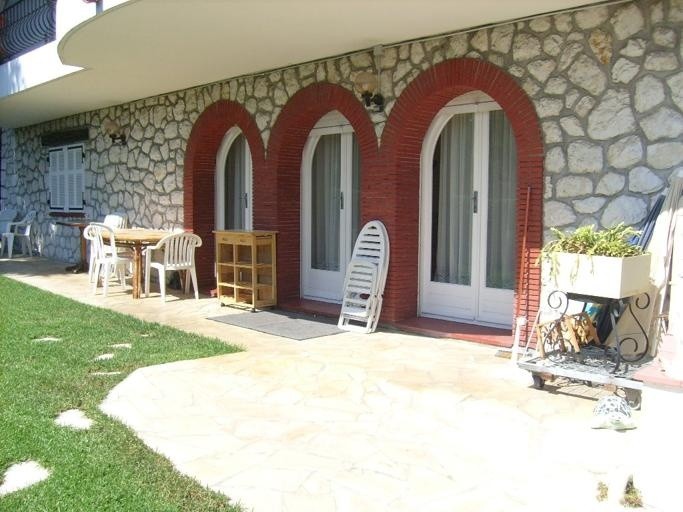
512;340;651;410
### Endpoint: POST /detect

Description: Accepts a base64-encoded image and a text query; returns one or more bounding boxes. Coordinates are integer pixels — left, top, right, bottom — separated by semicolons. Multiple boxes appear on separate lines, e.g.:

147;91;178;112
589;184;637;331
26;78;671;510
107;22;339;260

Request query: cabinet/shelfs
211;229;280;313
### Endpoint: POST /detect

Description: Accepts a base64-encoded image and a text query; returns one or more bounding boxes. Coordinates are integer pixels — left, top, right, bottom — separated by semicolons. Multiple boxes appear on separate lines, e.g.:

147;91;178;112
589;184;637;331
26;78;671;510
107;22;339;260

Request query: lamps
350;72;384;107
101;121;126;144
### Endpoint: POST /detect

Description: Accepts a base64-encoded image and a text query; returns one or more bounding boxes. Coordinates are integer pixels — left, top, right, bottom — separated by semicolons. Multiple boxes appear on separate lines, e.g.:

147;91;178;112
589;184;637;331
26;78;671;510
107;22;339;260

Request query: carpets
205;306;349;343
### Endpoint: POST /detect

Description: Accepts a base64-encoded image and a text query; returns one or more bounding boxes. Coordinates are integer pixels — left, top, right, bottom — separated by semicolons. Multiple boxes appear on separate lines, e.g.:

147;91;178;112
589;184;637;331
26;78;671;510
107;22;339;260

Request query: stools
535;308;601;361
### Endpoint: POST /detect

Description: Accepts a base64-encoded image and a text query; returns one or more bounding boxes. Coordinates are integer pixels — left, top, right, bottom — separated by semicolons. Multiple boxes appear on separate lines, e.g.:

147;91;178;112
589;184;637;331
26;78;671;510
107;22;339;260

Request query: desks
55;220;100;274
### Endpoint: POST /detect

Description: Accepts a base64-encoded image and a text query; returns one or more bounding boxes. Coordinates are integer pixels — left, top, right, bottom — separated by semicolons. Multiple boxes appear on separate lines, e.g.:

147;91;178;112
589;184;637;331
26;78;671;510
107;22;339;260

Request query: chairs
0;209;18;258
83;212;203;302
0;210;36;258
335;216;393;335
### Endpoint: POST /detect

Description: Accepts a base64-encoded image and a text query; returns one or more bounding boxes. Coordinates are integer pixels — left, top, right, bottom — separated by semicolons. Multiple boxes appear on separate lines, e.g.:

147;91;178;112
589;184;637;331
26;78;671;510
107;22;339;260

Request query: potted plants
532;218;655;301
